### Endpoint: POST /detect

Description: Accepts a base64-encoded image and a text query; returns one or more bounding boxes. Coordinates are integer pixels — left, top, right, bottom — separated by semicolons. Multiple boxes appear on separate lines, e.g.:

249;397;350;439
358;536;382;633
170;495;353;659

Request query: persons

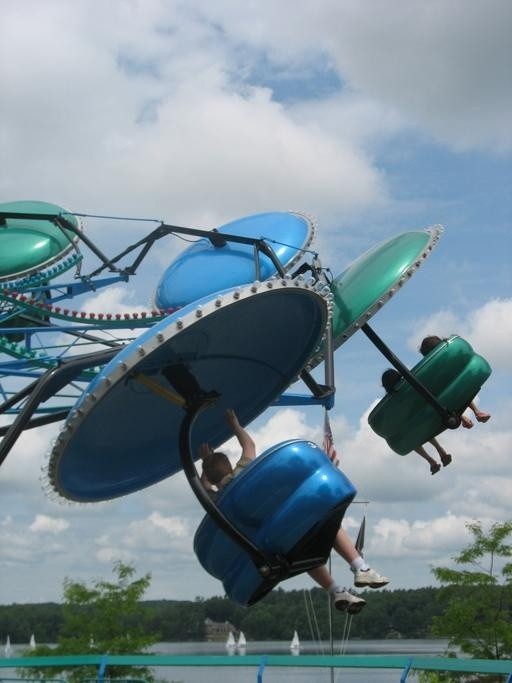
196;405;388;613
380;366;456;475
418;334;492;430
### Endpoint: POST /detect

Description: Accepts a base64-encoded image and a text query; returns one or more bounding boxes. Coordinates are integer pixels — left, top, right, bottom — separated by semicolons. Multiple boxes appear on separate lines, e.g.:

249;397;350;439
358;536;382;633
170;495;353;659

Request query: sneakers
332;587;369;615
475;412;491;423
430;463;442;475
352;568;391;589
461;420;474;430
440;454;453;468
